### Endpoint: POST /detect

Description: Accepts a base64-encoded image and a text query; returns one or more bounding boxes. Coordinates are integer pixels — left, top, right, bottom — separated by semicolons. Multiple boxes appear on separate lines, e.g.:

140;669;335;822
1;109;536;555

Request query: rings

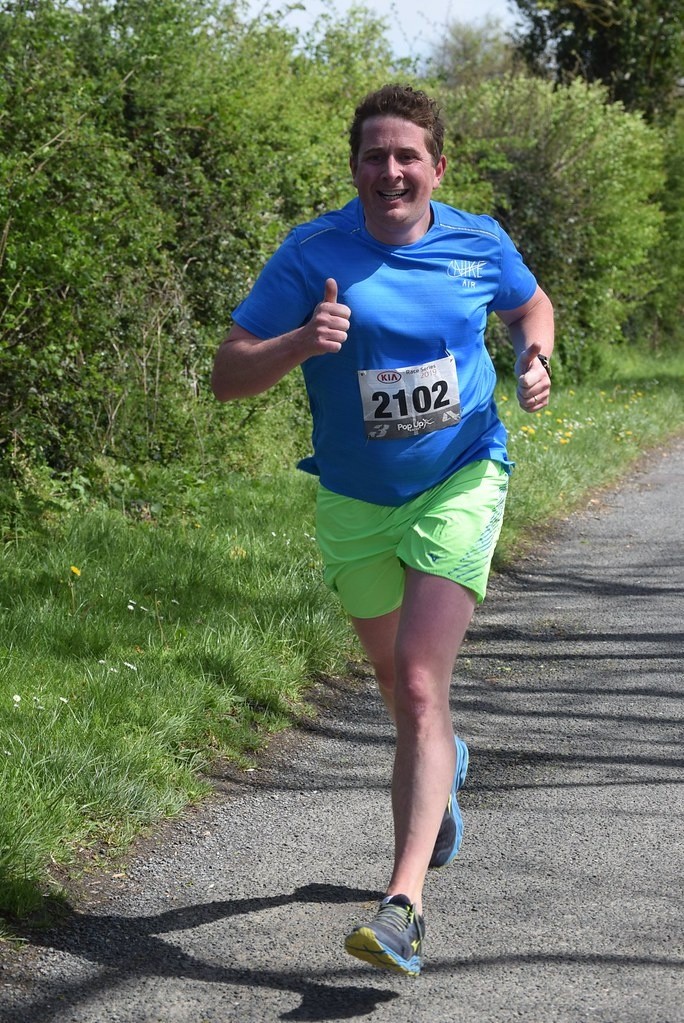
534;396;537;406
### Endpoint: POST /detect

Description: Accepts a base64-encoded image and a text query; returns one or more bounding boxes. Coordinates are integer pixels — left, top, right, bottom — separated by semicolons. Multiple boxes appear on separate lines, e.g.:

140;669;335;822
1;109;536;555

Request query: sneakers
427;735;468;870
344;893;424;975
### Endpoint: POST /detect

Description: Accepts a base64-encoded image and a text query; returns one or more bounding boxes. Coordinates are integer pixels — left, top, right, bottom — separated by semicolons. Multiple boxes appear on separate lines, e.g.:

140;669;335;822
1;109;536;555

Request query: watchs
537;354;551;380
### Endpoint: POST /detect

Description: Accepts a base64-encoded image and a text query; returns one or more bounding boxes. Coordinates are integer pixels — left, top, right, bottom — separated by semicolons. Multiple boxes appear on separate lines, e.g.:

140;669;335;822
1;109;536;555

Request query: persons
212;86;554;978
